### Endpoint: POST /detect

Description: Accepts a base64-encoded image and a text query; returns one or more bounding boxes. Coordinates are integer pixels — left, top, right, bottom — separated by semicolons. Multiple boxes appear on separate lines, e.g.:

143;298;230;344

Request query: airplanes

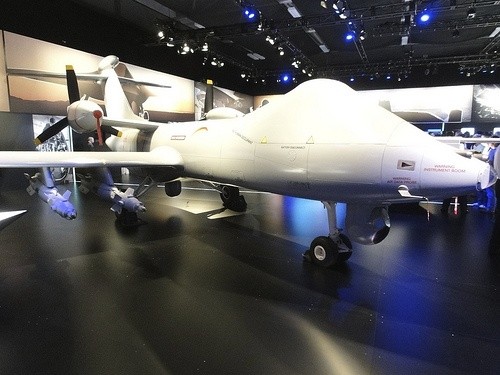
0;56;499;270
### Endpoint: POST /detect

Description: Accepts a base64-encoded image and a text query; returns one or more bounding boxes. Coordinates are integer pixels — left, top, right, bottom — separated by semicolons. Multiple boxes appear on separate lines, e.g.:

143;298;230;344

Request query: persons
428;130;500;213
